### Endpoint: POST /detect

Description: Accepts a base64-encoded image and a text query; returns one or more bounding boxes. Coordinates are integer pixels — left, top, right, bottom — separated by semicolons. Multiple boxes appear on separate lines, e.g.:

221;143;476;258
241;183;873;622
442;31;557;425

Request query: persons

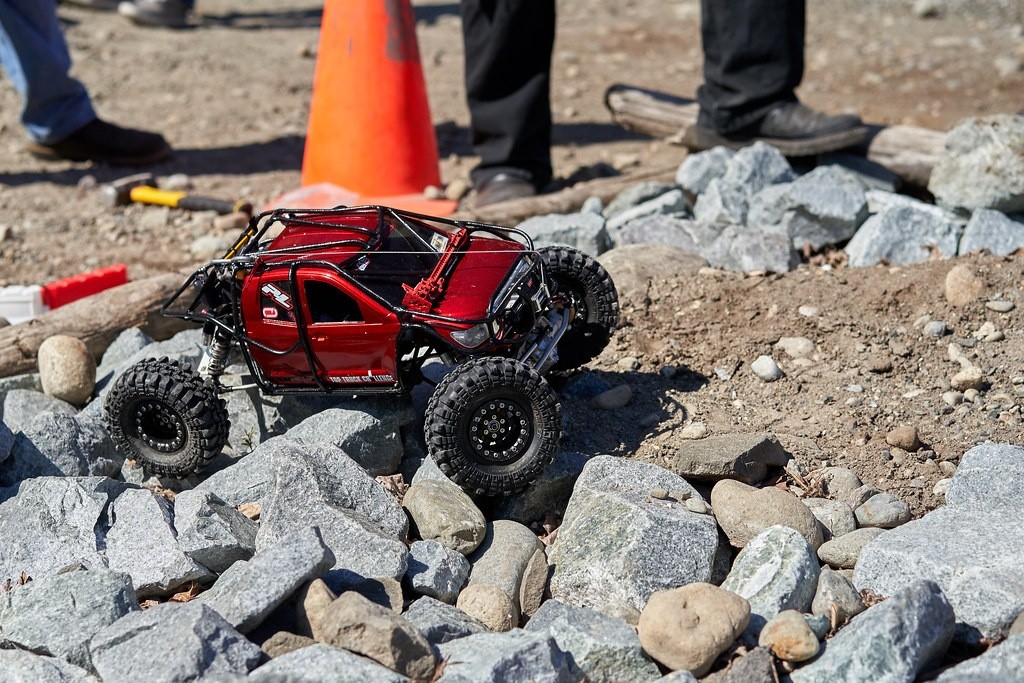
0;0;197;169
459;0;867;207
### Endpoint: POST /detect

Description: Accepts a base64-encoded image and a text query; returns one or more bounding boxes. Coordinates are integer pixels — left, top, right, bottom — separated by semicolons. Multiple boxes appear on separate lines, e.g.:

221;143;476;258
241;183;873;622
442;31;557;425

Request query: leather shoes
681;84;870;155
468;173;570;224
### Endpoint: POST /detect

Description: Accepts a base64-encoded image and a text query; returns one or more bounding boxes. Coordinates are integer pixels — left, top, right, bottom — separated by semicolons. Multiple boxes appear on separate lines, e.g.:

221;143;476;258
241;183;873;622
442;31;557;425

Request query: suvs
101;204;619;501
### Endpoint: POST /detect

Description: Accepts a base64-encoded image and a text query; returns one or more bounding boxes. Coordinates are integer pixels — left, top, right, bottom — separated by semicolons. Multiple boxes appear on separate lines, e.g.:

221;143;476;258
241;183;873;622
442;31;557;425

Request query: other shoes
28;117;172;167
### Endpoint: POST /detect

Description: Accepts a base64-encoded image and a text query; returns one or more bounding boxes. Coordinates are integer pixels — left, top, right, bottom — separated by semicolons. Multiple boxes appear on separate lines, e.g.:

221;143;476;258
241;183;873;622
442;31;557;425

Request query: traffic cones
249;1;461;221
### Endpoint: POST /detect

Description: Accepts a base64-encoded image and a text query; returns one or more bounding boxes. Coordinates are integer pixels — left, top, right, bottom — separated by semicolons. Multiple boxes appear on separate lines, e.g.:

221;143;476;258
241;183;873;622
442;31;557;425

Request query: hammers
100;171;253;215
